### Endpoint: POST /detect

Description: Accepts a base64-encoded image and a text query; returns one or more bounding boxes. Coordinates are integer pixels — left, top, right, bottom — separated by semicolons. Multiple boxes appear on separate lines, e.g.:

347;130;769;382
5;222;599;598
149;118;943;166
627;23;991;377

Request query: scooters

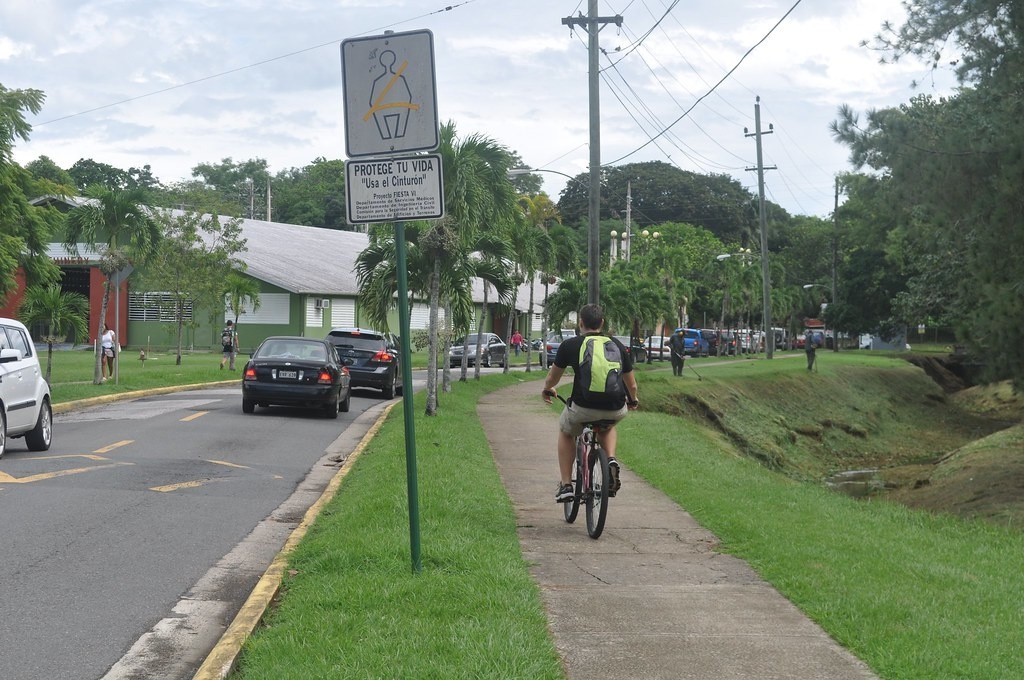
522;338;543;352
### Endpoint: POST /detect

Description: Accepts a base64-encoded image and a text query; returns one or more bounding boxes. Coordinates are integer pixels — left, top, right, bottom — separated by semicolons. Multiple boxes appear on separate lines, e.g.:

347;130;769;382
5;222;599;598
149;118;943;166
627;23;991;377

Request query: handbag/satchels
111;341;121;353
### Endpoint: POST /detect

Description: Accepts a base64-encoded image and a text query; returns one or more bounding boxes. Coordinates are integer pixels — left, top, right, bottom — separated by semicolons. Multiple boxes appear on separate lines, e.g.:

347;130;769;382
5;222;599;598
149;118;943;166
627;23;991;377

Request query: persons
100;322;116;382
668;329;685;377
542;304;638;501
511;329;523;357
805;329;816;371
220;320;240;371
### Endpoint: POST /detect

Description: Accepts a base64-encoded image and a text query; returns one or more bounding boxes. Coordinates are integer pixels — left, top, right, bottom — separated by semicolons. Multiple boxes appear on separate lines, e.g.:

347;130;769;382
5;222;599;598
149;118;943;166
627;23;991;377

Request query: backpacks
573;334;626;405
222;329;233;346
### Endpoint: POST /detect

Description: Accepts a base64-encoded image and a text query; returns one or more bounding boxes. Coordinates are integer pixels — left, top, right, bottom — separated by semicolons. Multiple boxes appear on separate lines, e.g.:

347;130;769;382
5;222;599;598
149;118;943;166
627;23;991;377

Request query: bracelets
236;347;239;348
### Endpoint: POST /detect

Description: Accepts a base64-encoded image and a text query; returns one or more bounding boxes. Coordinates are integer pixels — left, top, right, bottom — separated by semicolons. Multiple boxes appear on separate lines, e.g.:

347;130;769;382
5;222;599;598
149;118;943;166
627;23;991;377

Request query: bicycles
545;389;638;540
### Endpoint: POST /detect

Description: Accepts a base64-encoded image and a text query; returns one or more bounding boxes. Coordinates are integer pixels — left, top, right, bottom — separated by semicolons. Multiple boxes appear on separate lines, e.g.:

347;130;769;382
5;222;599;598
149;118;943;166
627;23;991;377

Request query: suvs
324;328;402;400
674;328;709;357
771;327;789;351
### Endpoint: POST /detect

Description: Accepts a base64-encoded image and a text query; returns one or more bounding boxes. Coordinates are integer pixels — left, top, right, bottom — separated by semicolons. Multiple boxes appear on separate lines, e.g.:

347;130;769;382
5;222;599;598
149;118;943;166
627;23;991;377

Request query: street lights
506;169;600;304
804;284;840;352
716;253;773;359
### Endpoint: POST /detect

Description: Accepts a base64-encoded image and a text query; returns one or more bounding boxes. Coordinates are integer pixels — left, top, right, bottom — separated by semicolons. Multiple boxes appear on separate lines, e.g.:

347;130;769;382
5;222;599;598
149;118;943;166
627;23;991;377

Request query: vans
547;329;576;339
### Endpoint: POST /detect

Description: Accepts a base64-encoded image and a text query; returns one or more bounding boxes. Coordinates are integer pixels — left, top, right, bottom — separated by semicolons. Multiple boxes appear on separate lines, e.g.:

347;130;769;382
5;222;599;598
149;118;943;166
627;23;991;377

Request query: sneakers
554;481;575;501
606;457;621;493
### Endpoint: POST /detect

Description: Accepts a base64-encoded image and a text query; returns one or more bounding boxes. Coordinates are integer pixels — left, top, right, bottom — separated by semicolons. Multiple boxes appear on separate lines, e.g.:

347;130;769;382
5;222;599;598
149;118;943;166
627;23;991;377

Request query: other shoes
102;376;107;382
108;375;113;380
219;363;223;370
230;369;236;371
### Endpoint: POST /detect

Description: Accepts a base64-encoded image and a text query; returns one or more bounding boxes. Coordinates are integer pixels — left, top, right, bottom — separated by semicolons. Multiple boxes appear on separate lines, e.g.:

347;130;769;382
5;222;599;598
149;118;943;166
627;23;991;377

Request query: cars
539;335;577;365
0;318;53;460
721;330;742;355
613;335;648;363
451;333;506;367
642;336;671;360
794;335;807;348
736;328;768;352
806;330;833;349
241;335;352;419
702;329;728;355
